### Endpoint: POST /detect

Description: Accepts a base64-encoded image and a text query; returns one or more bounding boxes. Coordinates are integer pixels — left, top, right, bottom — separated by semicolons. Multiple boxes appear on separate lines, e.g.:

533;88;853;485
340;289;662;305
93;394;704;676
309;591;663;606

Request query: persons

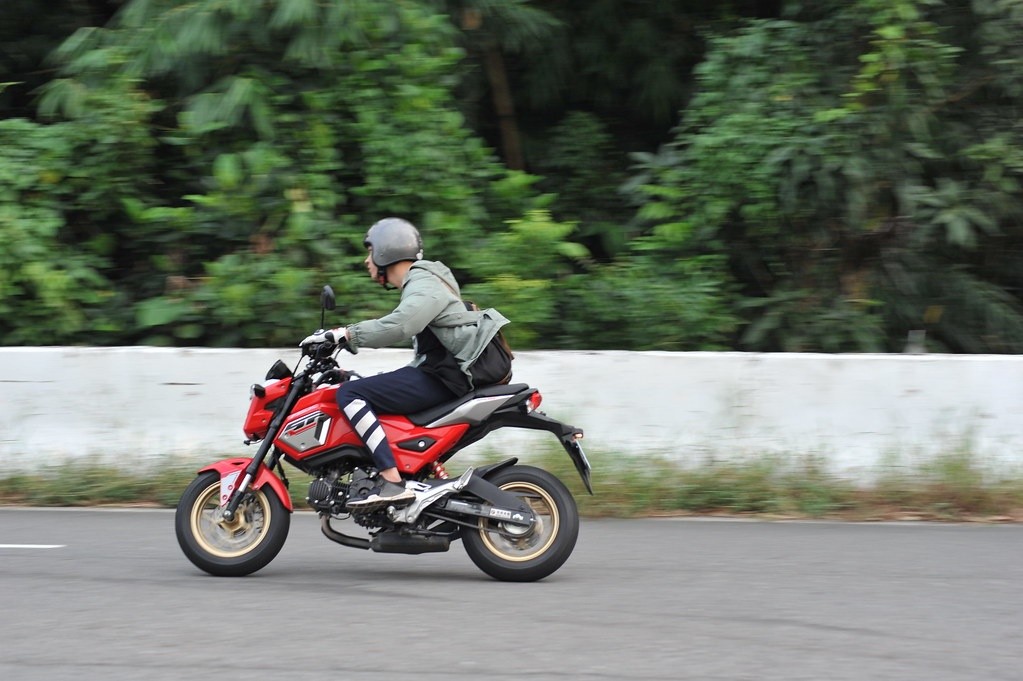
299;217;511;510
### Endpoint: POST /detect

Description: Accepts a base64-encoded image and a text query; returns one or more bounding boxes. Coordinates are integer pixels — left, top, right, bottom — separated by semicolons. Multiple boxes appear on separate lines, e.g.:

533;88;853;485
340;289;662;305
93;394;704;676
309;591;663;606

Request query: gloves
299;327;349;347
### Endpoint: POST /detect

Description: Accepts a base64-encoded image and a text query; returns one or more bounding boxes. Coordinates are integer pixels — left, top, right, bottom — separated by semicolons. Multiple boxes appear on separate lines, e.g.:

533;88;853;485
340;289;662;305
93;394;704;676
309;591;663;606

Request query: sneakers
346;474;416;512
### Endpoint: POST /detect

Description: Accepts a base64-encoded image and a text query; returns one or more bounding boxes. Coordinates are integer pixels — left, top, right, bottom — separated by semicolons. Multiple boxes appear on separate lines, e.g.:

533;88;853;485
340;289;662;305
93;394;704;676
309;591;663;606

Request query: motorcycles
176;284;593;583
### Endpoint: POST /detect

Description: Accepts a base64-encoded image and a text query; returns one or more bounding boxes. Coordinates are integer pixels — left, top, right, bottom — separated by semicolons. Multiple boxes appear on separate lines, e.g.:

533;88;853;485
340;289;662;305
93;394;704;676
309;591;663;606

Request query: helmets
362;216;423;268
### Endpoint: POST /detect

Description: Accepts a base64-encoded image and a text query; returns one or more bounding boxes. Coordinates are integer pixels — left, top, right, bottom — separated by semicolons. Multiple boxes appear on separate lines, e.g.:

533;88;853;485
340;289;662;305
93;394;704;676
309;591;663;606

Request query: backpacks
404;263;514;389
